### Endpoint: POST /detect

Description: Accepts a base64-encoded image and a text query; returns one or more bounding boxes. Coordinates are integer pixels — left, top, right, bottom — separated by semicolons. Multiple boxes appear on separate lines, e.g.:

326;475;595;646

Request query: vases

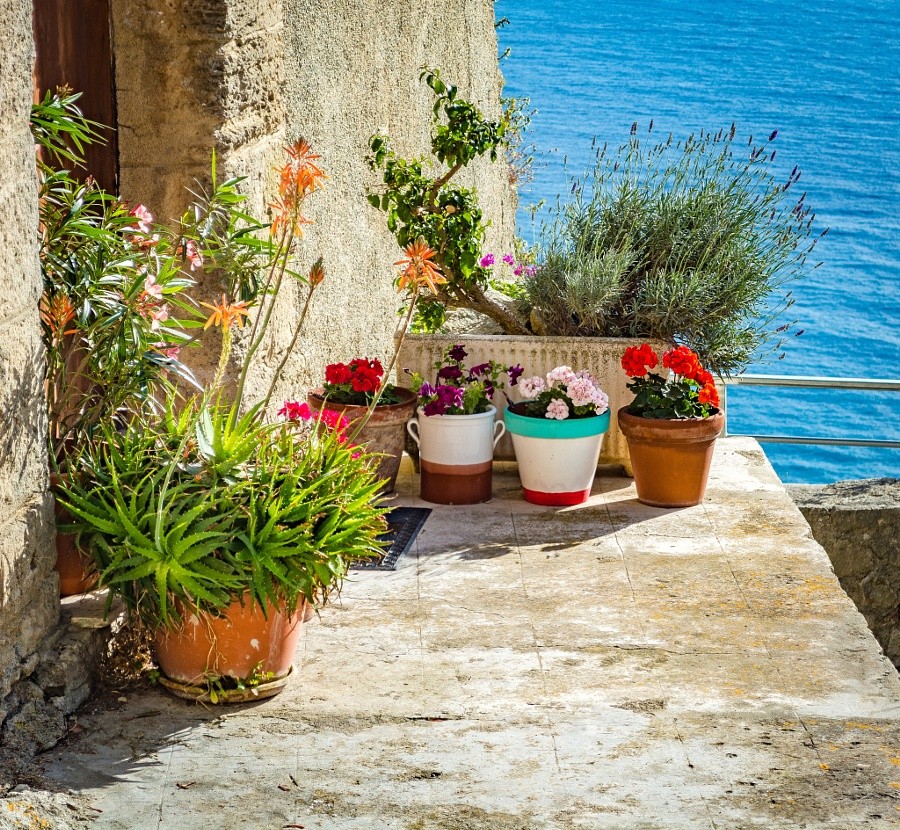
617;406;726;506
503;400;610;506
308;386;417;496
407;404;505;506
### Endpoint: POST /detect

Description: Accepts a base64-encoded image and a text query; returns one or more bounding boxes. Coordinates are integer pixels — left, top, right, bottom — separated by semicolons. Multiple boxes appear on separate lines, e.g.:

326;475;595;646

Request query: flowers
519;365;610;419
321;358;406;405
481;253;537;299
621;344;722;419
403;342;523;417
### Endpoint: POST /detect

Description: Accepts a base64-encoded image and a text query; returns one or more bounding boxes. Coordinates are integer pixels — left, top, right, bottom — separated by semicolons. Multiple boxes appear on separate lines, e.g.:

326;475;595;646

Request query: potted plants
54;392;394;699
362;62;832;480
30;83;324;594
